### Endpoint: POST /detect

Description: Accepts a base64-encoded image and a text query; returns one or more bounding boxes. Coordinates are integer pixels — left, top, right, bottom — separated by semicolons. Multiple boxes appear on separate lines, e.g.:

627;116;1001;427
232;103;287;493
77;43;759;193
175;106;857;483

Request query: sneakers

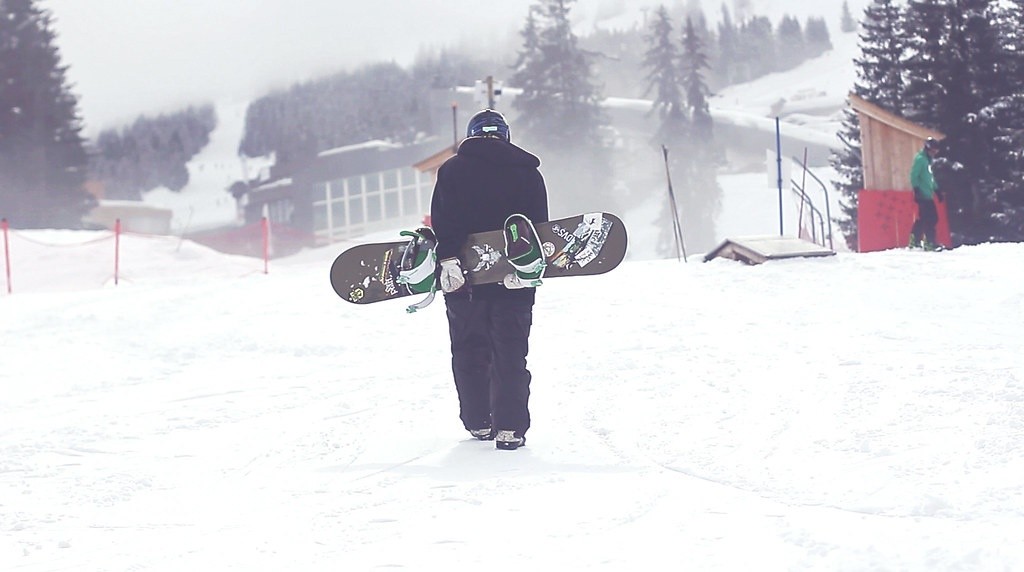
496;429;526;449
924;242;942;252
471;428;494;440
909;233;922;248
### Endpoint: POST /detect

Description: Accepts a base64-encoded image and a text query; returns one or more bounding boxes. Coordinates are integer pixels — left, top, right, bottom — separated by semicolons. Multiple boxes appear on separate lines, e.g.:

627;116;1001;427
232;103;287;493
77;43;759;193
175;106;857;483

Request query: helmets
925;137;936;148
466;109;510;142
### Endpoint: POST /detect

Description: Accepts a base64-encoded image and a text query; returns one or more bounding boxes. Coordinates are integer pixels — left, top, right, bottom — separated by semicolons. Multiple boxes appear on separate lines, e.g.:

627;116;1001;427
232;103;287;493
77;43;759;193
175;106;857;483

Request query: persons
908;136;944;252
430;110;549;451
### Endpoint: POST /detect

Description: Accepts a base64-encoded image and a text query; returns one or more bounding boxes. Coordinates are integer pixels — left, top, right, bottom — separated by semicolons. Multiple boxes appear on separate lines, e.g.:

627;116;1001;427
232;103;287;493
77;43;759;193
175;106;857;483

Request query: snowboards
330;210;629;303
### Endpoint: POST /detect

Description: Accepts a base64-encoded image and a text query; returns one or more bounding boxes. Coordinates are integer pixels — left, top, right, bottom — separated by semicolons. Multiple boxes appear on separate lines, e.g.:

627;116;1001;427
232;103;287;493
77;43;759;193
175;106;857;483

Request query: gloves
915;192;920;202
937;192;943;201
434;255;472;297
504;274;539;289
